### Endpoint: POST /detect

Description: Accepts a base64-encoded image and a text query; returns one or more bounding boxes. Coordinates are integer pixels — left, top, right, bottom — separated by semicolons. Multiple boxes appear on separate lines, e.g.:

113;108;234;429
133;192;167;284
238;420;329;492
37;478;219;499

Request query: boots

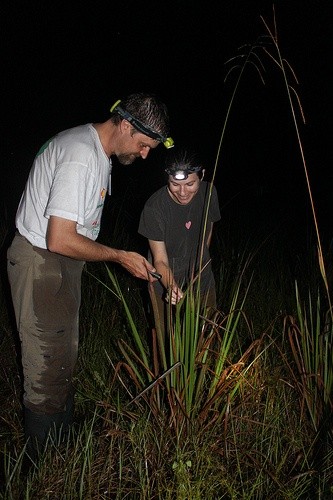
21;407;65;473
62;397;81;438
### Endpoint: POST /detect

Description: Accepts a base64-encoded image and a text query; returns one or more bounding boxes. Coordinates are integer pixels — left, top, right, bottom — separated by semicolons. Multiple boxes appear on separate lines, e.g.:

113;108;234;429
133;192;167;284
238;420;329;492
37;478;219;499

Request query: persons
6;89;178;474
137;148;221;342
